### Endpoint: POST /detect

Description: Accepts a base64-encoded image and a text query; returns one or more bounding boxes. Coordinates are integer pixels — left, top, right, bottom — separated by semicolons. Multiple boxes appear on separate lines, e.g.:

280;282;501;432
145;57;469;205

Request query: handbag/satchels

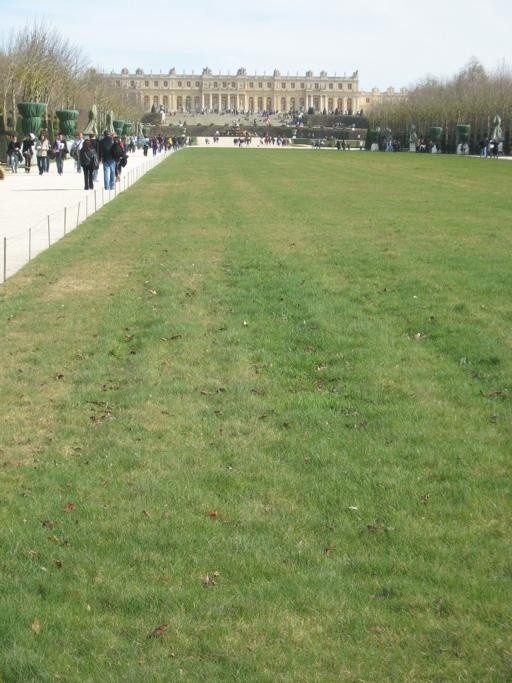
111;143;123;163
48;150;55;159
120;158;127;168
91;154;99;170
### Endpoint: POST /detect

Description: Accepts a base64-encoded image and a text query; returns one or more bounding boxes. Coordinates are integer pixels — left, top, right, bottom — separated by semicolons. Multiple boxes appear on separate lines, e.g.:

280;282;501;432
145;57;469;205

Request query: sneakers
115;174;120;182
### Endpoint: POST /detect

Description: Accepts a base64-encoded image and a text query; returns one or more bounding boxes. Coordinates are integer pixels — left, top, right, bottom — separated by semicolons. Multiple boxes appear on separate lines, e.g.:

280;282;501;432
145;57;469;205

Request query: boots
24;166;30;173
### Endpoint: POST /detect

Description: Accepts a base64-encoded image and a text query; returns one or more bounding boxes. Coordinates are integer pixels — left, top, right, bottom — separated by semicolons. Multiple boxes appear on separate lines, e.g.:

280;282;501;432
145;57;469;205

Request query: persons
205;136;219;145
169;108;301;125
416;139;441;153
335;139;401;152
456;142;469;154
7;132;83;176
121;132;192;156
81;131;124;191
234;134;284;146
311;141;321;152
479;138;499;159
